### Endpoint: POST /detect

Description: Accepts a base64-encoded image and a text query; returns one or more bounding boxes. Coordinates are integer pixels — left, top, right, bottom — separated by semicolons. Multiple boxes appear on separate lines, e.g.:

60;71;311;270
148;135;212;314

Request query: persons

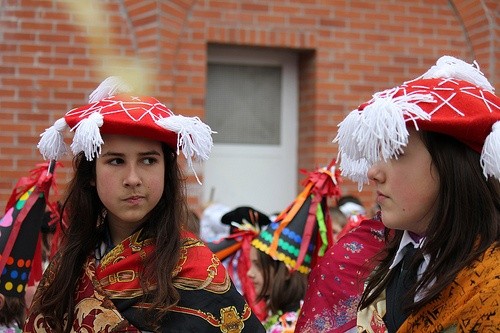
0;158;395;332
331;55;500;333
24;76;267;333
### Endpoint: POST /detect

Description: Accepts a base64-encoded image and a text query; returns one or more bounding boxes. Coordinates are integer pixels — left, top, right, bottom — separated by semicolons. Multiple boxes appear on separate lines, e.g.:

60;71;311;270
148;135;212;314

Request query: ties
391;243;425;333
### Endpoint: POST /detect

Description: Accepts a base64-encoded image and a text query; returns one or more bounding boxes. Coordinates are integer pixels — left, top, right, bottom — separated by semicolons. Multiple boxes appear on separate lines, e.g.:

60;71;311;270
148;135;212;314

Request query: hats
0;158;57;299
221;207;272;234
330;54;500;193
249;159;338;275
36;76;217;185
